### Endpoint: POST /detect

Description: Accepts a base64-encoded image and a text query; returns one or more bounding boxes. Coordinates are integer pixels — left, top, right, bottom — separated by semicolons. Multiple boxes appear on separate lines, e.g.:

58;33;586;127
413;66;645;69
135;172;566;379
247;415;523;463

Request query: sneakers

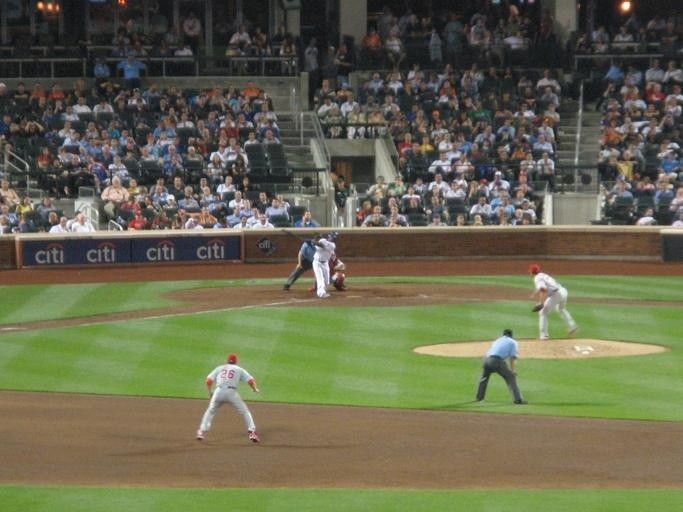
476;395;483;401
248;431;260;443
307;283;347;299
539;327;577;340
196;430;205;439
283;284;290;291
514;399;528;404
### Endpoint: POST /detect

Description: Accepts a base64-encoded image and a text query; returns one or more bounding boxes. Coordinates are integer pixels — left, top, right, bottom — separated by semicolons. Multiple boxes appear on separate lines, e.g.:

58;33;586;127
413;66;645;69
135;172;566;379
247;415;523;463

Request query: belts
219;386;237;389
553;288;558;292
486;355;501;359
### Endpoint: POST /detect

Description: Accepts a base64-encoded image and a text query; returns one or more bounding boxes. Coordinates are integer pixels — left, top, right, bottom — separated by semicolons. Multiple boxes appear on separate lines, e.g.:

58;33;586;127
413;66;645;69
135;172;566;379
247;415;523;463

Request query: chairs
42;89;292;196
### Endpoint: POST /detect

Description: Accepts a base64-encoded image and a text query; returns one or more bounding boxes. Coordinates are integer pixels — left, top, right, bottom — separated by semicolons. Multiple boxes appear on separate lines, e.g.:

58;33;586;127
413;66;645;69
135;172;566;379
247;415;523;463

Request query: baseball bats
282;229;306;241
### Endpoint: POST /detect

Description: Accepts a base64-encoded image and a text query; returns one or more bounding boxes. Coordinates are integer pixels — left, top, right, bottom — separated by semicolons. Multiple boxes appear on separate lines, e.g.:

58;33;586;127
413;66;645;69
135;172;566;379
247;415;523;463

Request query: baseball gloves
533;304;544;312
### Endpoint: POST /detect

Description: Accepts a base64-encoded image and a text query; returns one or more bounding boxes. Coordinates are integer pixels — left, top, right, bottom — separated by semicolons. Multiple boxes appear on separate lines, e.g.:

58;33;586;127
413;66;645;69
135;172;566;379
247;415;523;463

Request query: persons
280;235;317;291
193;354;262;443
1;2;681;233
473;328;526;407
310;232;336;300
528;264;580;340
309;254;350;292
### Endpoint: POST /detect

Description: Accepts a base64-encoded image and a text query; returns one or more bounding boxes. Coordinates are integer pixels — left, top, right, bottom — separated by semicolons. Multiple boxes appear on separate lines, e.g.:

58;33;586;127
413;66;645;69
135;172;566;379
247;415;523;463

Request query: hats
527;263;540;273
494;171;501;176
227;355;236;363
504;329;512;336
136;210;143;216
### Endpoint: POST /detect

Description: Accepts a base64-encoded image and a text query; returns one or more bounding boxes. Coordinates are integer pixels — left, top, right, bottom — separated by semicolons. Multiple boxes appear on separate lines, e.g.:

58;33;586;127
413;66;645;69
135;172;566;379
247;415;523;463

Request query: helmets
328;231;339;240
